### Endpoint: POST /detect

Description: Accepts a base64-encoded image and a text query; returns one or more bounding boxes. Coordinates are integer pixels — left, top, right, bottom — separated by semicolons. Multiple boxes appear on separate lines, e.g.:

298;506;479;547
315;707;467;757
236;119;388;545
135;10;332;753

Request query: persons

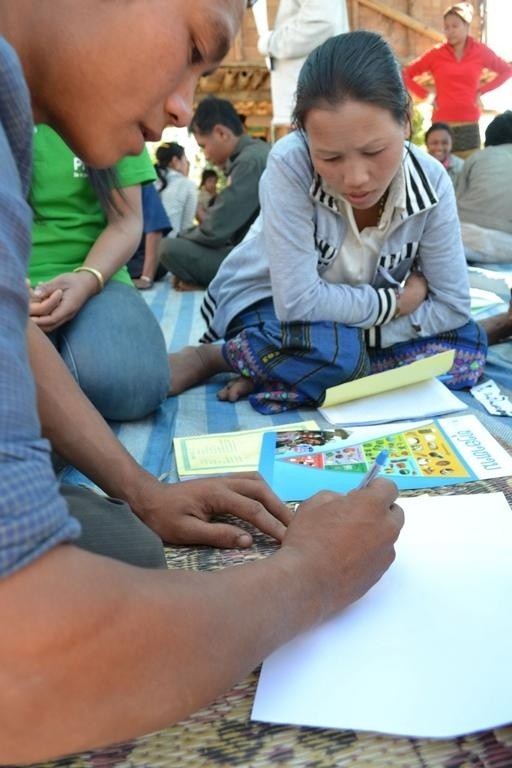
3;1;510;768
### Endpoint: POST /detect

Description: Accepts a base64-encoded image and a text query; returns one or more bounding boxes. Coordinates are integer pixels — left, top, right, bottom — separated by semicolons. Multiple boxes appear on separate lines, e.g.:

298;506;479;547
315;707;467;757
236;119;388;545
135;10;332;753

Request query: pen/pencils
356;450;388;491
